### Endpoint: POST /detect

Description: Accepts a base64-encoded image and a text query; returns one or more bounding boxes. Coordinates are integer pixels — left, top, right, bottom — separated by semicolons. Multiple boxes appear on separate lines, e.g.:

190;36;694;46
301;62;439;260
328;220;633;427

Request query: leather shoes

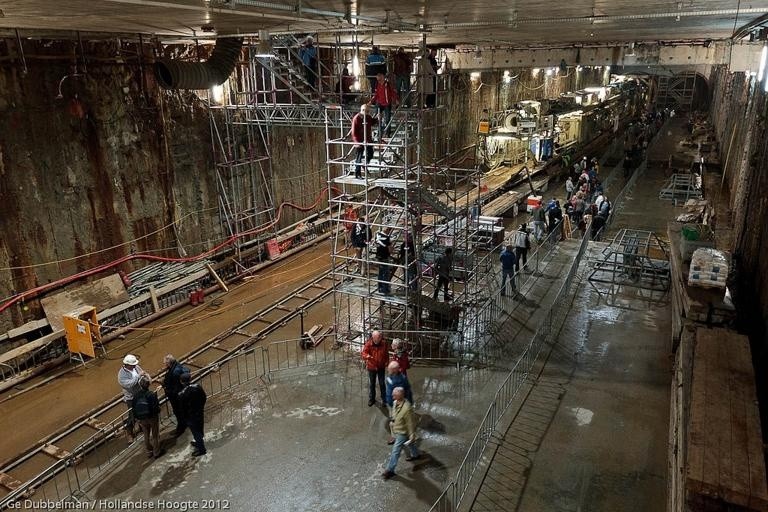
190;442;201;456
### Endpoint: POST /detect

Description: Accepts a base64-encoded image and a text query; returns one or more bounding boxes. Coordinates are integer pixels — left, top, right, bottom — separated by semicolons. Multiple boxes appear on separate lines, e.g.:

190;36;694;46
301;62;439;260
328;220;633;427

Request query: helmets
122;354;138;366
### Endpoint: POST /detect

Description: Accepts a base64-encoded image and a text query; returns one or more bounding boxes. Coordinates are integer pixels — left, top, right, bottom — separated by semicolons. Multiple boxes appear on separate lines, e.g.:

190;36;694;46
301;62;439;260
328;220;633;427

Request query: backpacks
135;390;153;420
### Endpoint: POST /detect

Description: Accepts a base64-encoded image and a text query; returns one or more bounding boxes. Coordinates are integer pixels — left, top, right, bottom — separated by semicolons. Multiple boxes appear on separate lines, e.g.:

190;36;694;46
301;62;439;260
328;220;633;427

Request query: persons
299;34;677;479
118;353;209;458
